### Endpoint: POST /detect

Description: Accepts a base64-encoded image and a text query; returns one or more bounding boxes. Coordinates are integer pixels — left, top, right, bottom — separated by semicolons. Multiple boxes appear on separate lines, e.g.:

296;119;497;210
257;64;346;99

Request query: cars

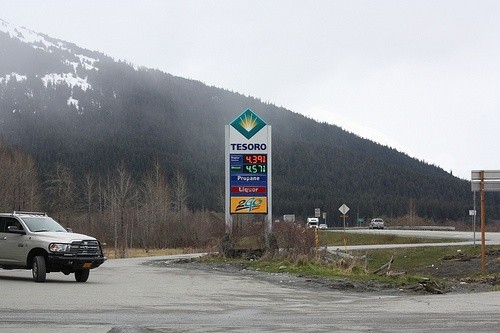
318;224;328;230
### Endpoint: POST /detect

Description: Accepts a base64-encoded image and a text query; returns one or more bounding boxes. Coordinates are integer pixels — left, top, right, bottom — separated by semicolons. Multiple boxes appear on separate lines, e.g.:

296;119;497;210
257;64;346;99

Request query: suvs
368;218;384;229
0;210;107;283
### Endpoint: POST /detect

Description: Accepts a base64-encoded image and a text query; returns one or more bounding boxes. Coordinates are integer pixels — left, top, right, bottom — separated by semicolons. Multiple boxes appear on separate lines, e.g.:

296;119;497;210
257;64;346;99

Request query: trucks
307;217;319;230
